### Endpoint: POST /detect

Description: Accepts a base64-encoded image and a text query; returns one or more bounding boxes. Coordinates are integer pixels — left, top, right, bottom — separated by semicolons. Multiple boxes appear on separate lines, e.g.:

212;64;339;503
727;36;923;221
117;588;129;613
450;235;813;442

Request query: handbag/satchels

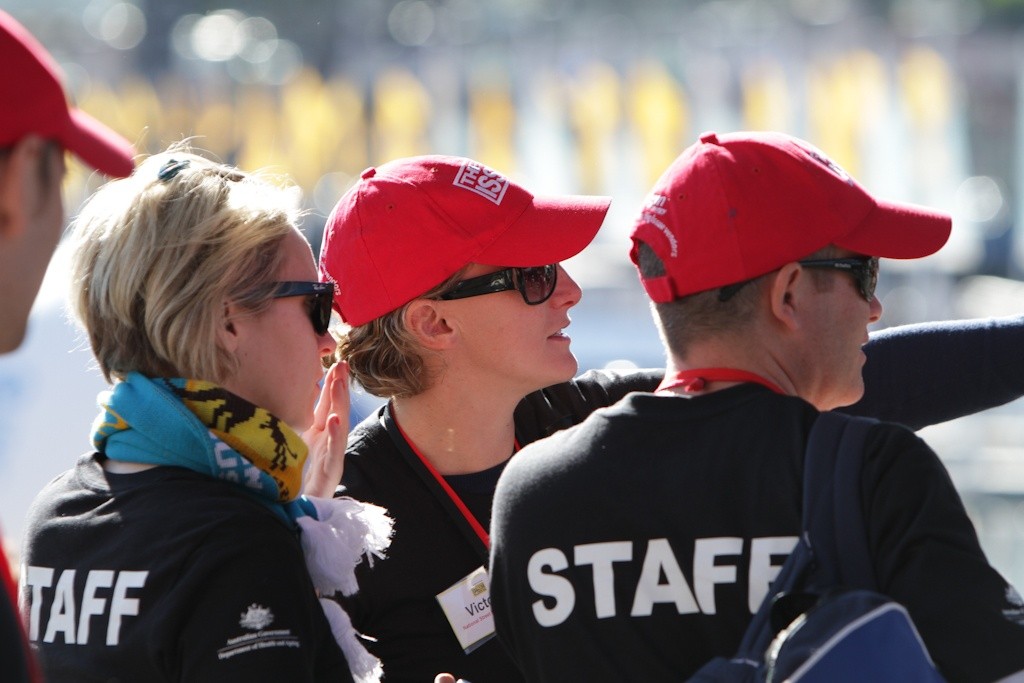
692;413;946;682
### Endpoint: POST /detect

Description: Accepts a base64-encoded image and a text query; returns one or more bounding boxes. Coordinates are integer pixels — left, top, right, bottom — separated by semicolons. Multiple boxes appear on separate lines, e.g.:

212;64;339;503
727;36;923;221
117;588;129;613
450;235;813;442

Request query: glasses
722;254;881;305
430;261;559;306
238;283;339;336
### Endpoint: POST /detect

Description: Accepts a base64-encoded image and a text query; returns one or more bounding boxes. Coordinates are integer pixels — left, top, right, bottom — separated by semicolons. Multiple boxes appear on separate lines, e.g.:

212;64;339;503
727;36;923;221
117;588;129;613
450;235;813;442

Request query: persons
319;156;1024;683
19;147;394;682
0;9;1024;682
488;132;1024;681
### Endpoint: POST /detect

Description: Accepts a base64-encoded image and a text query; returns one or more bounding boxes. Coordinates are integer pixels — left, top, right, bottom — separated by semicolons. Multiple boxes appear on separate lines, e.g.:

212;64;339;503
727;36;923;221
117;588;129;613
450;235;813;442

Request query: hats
628;132;953;305
0;12;137;178
319;151;611;327
